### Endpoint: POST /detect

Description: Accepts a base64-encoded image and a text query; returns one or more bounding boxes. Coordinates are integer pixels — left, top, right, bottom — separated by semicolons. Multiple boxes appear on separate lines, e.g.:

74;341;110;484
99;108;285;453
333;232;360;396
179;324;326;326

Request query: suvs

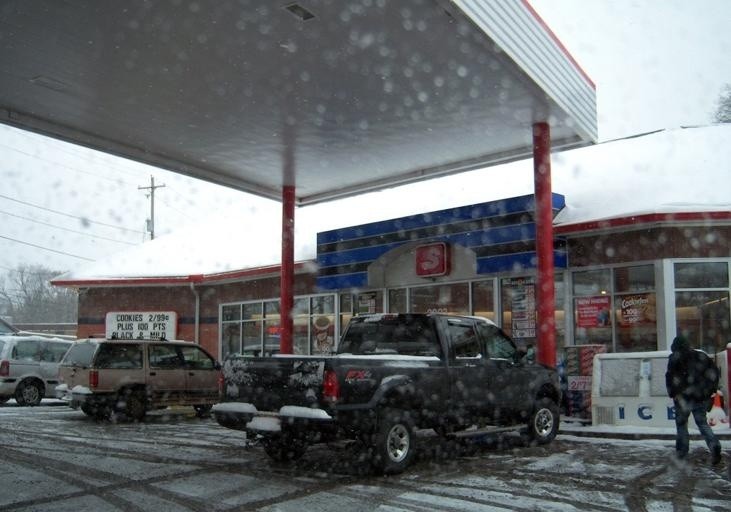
53;331;229;423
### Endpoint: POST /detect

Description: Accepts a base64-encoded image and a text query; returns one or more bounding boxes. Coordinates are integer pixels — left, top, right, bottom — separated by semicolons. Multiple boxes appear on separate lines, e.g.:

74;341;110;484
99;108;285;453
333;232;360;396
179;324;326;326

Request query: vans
0;334;76;409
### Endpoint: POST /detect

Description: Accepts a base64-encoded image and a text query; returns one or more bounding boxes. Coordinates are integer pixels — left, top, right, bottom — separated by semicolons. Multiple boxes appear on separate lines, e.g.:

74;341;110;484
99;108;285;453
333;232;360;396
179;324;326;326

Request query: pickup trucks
207;310;564;480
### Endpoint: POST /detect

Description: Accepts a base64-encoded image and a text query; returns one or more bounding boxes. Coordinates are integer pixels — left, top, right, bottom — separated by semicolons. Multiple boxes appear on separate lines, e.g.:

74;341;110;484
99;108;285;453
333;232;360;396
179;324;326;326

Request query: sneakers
711;445;721;465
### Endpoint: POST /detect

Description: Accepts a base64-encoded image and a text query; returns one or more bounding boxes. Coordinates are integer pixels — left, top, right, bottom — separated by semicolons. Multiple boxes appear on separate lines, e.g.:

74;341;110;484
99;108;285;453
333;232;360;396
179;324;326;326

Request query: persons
666;335;721;464
